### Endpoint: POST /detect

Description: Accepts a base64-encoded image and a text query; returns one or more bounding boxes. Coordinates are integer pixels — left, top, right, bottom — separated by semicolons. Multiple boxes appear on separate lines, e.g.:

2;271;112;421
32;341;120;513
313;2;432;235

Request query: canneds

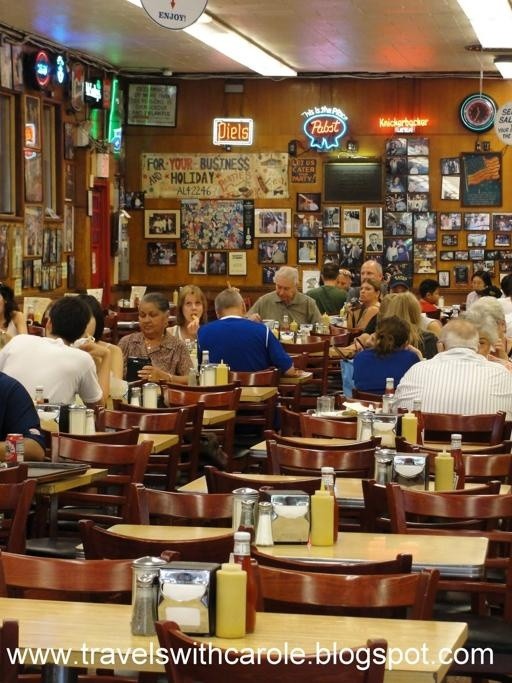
6;433;24;467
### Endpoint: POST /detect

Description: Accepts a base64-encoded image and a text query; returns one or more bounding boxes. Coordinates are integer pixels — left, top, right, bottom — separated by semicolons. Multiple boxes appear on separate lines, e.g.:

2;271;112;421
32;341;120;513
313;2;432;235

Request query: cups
299;323;313;336
316;394;335;416
382;394;395;415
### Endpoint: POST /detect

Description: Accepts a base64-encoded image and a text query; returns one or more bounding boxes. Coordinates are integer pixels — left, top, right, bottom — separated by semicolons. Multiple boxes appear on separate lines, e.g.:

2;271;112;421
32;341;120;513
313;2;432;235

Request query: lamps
126;0;297;77
457;0;512;80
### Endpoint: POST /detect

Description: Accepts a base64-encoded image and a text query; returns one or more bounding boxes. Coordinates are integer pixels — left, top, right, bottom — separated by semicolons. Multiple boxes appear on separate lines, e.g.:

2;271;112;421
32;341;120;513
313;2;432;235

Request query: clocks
458;93;498;134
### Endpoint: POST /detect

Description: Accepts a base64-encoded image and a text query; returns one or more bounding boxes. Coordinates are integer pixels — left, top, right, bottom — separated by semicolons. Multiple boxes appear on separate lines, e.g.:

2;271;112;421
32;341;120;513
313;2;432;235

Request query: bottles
131;386;142;408
434;447;455;493
438;296;445;310
307;466;339;546
447;433;466;492
231;486;277;547
67;403;88;435
339;306;346;317
356;411;374;444
372;449;399;486
397;399;424;448
173;289;180;304
269;314;298;346
142;383;161;410
188;350;228;387
135;293;140;308
25;305;36;333
227;531;259;635
216;552;249;639
35;385;45;405
314;311;331;335
83;407;97;436
184;337;192;352
384;377;395;395
124;556;166;637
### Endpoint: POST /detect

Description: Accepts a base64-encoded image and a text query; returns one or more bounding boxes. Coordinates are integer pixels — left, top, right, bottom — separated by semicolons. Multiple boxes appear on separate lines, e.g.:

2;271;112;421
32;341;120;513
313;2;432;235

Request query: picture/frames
0;85;77;292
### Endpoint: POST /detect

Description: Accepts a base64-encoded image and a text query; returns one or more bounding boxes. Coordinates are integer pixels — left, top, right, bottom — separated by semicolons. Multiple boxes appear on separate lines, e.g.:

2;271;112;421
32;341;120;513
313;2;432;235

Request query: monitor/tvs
322;162;384;204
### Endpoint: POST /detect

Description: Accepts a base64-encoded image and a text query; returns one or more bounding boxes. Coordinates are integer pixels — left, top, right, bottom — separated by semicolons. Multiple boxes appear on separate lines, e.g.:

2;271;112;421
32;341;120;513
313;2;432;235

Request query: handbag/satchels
339;360;355;398
184;433;229;469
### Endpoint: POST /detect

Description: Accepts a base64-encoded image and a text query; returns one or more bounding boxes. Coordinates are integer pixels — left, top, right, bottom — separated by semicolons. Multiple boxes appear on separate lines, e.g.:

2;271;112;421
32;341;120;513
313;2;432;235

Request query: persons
0;259;512;518
130;138;512;297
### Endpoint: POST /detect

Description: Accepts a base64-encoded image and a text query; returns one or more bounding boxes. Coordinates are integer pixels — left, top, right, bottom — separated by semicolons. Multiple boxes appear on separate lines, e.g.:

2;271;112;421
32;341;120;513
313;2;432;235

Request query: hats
388;273;410;289
